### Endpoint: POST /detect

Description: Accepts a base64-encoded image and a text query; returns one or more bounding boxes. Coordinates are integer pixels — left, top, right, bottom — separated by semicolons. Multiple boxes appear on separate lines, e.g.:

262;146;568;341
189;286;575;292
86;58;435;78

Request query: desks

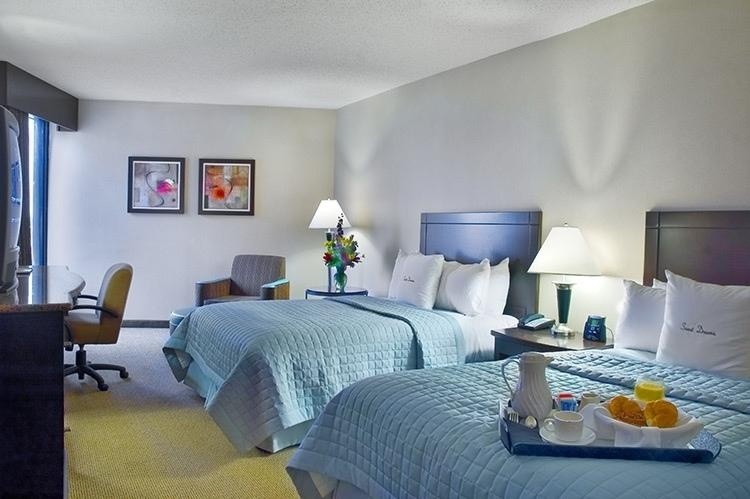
304;285;369;300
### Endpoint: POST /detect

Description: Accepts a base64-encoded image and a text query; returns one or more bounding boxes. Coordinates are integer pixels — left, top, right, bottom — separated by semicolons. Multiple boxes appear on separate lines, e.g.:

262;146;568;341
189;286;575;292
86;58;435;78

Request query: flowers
322;216;366;288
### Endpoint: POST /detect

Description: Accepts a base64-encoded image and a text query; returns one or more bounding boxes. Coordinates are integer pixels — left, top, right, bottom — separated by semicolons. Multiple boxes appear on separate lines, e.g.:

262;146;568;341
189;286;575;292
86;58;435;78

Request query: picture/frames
126;155;187;216
196;157;259;216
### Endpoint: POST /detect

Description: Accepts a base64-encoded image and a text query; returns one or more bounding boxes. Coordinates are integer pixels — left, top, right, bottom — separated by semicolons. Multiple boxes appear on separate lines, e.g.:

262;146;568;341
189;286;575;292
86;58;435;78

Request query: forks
507;409;520;424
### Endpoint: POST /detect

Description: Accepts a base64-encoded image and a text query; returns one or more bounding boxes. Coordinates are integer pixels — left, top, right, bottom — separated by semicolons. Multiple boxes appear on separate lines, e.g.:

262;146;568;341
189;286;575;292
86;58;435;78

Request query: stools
169;307;196;340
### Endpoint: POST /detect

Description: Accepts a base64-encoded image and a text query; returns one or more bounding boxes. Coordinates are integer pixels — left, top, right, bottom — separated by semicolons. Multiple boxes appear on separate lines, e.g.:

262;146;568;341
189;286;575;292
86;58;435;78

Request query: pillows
385;245;511;319
613;277;669;354
649;276;670;292
653;264;750;380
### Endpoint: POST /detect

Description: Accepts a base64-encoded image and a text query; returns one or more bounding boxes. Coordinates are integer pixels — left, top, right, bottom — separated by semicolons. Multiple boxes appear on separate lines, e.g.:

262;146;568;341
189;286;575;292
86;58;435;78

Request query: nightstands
490;323;616;365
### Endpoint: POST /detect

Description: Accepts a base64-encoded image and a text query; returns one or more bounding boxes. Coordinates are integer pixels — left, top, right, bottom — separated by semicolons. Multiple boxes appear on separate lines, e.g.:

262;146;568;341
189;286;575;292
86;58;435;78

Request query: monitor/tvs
0;105;23;293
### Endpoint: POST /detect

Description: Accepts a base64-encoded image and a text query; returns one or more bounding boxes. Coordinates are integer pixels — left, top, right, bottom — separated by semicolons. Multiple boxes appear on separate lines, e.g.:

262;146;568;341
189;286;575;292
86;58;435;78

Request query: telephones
517;314;556;331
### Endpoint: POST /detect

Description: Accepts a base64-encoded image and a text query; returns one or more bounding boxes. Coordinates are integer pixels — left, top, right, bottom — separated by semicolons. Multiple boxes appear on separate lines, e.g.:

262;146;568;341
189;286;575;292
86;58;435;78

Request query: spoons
544;409;585;442
524;415;538;430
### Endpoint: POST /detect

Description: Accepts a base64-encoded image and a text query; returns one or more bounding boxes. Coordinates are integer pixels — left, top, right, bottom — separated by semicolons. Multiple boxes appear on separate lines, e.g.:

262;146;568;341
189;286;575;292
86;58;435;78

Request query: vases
333;264;348;293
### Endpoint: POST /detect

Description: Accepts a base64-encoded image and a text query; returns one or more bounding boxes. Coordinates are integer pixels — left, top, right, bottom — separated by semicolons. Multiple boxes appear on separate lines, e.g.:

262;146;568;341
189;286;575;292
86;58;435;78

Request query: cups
561;398;578;412
632;380;665;401
581;392;601;405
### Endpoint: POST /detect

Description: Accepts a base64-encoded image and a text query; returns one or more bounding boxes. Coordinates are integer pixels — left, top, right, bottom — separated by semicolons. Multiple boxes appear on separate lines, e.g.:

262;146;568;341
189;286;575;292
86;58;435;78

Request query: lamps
305;198;354;287
524;224;604;337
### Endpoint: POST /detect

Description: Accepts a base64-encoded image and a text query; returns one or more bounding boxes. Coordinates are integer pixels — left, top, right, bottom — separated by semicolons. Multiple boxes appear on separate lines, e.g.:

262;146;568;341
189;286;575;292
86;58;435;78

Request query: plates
539;425;595;446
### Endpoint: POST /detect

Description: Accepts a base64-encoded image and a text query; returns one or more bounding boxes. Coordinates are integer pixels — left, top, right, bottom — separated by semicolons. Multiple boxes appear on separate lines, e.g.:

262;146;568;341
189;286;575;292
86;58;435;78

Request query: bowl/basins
594;394;704;448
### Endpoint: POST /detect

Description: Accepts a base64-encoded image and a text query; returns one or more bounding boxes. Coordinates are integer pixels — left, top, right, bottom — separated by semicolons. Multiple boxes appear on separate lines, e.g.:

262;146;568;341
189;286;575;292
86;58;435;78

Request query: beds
160;207;545;458
283;208;750;499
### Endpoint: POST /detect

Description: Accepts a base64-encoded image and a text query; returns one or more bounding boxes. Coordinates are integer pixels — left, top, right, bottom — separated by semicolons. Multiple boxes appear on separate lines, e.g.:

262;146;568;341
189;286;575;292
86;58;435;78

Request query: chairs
61;262;135;393
191;253;291;307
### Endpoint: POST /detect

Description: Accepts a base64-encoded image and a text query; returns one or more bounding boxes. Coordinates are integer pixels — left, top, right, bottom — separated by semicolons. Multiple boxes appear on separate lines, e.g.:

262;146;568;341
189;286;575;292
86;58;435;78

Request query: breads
608;395;645;426
645;400;678;428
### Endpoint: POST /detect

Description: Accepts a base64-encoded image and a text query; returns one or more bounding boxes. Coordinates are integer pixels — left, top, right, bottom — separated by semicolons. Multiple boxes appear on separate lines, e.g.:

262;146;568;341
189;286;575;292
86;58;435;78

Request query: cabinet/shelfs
0;261;89;498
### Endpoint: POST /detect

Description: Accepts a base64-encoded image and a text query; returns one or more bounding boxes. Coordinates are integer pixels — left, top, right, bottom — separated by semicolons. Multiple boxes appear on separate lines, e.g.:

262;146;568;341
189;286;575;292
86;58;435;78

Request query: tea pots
501;352;556;420
